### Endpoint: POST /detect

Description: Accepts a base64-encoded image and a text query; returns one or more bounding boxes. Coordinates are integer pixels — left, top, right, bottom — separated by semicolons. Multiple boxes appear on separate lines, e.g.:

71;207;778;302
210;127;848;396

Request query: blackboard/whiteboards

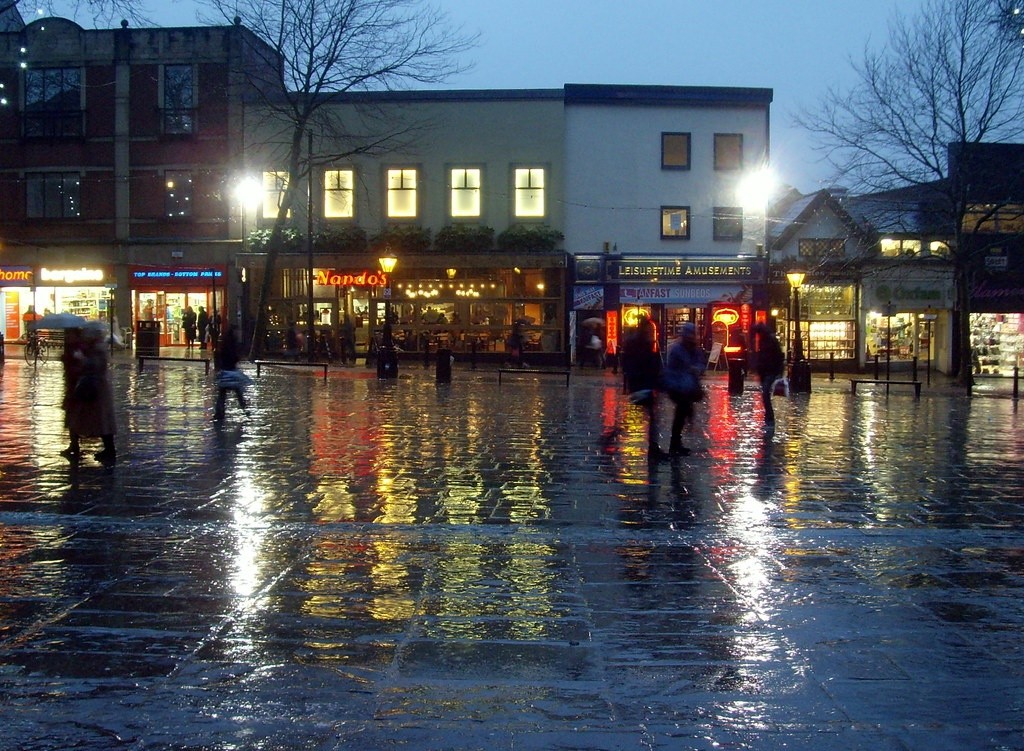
708;342;728;363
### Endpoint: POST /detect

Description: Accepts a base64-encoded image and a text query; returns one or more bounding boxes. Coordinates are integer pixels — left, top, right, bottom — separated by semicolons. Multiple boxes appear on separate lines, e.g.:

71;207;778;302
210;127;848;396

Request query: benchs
30;329;65;348
139;356;211;375
498;370;572;387
852;378;923;397
255;360;328;382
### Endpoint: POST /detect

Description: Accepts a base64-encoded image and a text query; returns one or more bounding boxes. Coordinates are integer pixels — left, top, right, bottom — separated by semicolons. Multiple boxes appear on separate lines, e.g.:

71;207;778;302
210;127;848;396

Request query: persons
508;318;528;369
624;319;704;458
24;304;50;331
360;306;368;319
167;304;248;413
578;323;607;369
382;304;461;348
61;328;117;461
753;322;782;421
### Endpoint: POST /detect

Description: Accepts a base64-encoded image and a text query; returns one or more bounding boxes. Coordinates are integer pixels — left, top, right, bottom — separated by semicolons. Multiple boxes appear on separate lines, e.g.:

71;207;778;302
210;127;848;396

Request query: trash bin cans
728;358;744;396
435;348;452;381
135;320;162;359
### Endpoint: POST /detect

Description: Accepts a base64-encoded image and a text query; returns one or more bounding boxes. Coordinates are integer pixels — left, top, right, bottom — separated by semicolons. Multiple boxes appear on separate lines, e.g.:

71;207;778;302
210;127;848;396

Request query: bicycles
21;330;49;366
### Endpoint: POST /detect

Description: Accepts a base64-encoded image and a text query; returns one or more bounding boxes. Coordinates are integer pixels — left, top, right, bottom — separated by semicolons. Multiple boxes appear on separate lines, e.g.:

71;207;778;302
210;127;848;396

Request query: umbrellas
32;313;85;328
583;317;605;327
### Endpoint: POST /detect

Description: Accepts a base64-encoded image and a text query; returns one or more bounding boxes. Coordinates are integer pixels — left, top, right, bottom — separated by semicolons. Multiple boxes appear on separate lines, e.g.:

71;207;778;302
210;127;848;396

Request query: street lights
786;259;811;394
377;242;398;379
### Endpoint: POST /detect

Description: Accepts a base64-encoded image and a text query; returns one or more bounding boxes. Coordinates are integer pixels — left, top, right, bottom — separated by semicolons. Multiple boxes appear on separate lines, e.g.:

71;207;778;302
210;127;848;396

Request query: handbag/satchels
508;335;520;347
215;367;242;391
694;379;704;404
512;347;519;357
772;377;790;397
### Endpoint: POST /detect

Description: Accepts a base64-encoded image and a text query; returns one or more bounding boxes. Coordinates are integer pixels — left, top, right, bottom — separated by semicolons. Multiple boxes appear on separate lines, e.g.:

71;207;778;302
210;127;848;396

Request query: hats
683;322;694;332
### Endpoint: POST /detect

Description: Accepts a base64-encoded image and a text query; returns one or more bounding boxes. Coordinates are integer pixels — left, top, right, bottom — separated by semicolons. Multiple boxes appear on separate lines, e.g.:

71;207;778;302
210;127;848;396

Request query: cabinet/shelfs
970;330;1001;374
67;298;97;316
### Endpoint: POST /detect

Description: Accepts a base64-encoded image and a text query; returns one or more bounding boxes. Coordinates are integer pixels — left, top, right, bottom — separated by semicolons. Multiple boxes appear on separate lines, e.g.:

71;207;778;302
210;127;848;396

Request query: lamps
446;267;456;279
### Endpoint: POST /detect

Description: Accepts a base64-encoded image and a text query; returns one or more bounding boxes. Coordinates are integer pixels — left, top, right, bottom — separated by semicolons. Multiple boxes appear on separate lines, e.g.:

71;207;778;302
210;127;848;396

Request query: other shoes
648;450;669;463
668;445;690;454
60;445;79;455
761;425;775;429
94;448;117;460
